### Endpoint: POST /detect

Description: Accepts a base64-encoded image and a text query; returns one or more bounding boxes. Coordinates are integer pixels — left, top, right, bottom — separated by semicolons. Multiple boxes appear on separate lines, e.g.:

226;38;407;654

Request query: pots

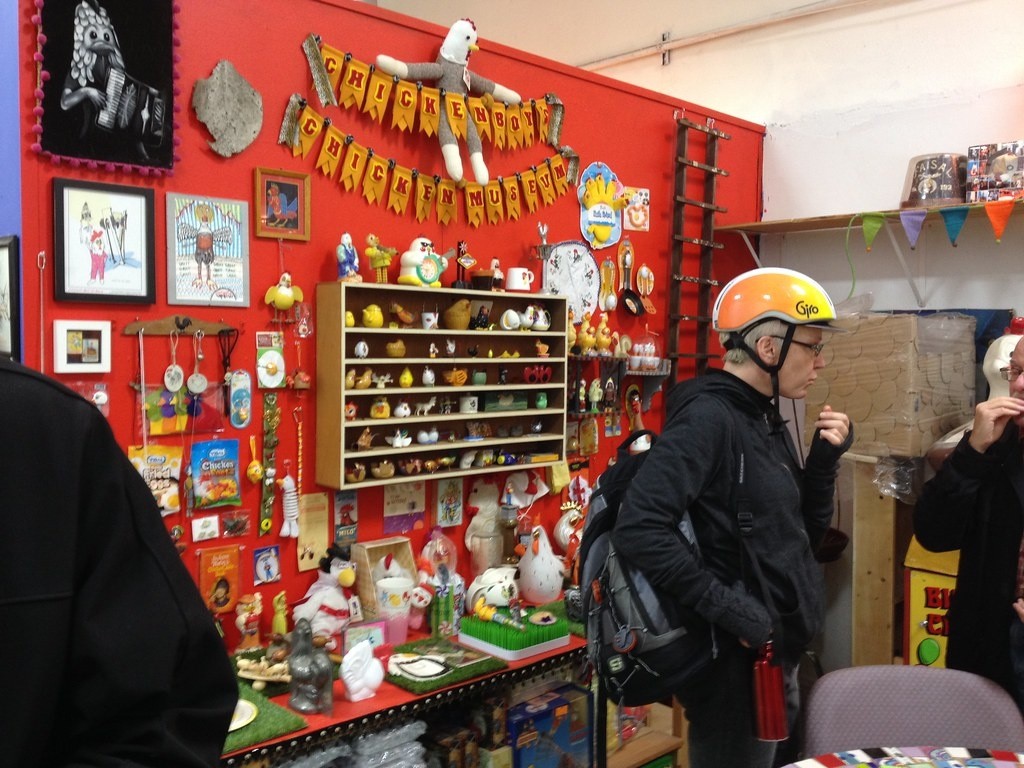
619;250;644;316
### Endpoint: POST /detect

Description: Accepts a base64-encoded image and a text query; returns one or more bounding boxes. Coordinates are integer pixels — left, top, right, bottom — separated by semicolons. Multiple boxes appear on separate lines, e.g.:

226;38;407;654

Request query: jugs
362;305;383;327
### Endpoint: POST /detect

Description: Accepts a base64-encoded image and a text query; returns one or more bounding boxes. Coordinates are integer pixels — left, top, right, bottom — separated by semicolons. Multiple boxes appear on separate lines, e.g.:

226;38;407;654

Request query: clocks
397;233;448;288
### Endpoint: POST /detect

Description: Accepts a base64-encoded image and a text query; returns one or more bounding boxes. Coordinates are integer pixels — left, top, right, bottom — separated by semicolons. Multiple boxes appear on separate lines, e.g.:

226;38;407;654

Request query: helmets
713;265;836;332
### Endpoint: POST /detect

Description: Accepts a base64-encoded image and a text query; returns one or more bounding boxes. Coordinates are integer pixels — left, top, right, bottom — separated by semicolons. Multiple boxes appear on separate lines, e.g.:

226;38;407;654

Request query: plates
228;699;258;730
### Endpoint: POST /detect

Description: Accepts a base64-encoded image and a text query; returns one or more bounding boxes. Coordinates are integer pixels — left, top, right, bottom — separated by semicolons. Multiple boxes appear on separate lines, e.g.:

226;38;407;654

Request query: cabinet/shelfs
316;284;581;496
203;606;715;768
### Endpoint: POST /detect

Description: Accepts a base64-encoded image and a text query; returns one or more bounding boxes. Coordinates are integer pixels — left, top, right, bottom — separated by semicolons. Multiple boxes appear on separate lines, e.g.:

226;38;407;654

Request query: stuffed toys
292;543;357;650
373;19;521;186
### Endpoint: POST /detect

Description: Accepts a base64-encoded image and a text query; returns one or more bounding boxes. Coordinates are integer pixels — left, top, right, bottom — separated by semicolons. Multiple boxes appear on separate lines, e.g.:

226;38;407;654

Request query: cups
460;396;478;413
629;356;660;371
376;577;413;643
534;392;548;409
470;532;504;574
472;372;486;384
505;267;535;293
421;313;439;328
470;269;494;290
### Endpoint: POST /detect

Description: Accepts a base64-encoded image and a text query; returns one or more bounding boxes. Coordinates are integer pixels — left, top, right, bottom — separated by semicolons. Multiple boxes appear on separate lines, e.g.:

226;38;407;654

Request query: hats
805;322;842;331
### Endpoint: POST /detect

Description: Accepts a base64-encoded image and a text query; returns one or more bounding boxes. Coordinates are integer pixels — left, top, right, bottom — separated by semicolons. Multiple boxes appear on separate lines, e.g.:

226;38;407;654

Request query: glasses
1000;366;1023;381
754;333;824;357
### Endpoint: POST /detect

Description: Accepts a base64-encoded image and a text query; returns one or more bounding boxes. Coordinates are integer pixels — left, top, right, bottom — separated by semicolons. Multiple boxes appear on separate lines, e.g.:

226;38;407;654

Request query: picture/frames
51;173;160;304
255;169;312;241
167;191;251;309
54;320;112;377
430;477;463;528
0;236;20;364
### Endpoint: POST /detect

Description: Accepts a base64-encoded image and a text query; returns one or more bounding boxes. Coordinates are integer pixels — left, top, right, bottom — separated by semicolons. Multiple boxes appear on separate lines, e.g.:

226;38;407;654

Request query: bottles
497;454;516;464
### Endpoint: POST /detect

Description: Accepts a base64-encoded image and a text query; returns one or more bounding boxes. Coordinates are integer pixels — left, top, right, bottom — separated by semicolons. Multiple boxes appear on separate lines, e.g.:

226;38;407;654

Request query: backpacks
578;388;749;747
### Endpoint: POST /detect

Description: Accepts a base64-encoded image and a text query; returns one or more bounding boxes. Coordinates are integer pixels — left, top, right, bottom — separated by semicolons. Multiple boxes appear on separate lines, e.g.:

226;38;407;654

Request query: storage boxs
813;314;978;458
505;685;596;768
899;142;1024;211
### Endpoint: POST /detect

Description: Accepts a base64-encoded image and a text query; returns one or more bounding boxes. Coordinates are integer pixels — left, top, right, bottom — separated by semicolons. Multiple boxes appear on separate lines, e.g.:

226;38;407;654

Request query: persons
235;592;263;650
913;337;1024;710
271;591;288;636
0;357;240;768
609;269;853;768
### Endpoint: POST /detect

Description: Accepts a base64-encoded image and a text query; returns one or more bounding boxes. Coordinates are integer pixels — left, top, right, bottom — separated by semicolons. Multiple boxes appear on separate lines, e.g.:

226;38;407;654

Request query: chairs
805;666;1024;757
